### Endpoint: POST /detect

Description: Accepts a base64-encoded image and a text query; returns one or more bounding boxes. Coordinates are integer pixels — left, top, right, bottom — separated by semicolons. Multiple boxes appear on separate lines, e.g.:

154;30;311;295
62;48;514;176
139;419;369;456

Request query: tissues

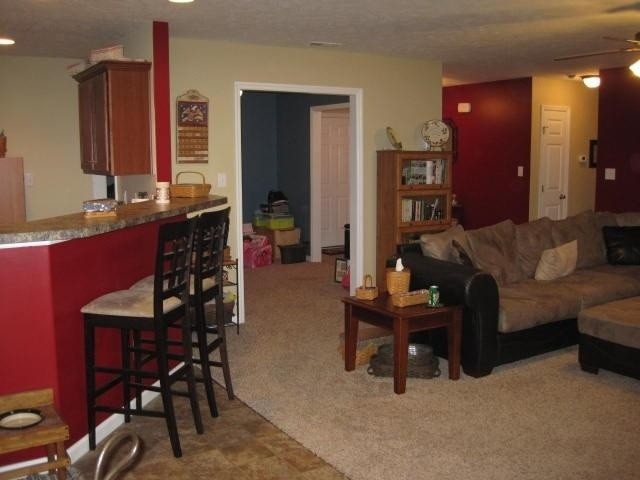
386;258;411;295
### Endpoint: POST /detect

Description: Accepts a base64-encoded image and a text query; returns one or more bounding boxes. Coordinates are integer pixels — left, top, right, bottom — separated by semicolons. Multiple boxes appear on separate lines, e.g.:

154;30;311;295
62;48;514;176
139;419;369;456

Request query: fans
547;35;640;62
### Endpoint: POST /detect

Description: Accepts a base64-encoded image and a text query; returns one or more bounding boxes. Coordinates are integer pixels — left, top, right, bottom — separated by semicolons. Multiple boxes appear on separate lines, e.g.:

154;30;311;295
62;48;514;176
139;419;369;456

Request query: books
403;159;444;184
401;198;443;222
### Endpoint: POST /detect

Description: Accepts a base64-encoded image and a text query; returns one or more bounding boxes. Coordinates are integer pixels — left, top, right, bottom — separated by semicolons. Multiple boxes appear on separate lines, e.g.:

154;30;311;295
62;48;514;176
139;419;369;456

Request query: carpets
192;260;640;479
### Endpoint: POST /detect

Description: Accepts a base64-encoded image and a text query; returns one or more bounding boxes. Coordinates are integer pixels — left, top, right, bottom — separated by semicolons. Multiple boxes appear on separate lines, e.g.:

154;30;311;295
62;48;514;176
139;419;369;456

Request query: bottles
134;192;148;200
156;182;170;203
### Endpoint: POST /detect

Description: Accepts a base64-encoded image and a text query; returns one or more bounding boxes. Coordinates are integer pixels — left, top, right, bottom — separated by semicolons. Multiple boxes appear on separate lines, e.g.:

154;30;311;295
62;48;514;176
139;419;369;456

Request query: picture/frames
590;139;598;167
334;257;348;282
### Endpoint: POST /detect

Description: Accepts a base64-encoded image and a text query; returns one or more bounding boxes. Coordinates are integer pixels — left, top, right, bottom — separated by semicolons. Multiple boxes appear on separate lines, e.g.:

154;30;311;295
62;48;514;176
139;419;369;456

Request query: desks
0;187;228;473
339;287;463;396
0;388;74;479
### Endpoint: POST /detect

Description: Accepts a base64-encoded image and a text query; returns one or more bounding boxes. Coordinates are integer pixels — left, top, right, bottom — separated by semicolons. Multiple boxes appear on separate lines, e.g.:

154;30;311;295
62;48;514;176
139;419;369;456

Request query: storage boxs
277;242;309;263
242;237;273;269
255;226;302;260
253;211;296;230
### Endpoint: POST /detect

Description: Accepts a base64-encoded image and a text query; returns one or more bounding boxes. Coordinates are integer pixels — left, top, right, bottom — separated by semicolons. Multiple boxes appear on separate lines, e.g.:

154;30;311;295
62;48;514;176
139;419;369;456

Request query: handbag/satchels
276;240;306;264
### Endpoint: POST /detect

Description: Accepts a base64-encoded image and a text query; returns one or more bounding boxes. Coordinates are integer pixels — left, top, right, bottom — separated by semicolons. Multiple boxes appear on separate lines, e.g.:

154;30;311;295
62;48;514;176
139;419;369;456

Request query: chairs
131;206;233;419
80;214;203;461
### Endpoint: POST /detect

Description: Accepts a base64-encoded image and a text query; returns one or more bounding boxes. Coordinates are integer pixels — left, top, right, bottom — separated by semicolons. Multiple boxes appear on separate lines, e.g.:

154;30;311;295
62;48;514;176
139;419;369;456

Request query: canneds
428;286;440;307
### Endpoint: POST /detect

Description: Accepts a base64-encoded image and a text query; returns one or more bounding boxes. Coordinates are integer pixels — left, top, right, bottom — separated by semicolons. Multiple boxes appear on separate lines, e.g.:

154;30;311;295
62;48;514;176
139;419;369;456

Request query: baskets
169;171;211;198
355;273;379;301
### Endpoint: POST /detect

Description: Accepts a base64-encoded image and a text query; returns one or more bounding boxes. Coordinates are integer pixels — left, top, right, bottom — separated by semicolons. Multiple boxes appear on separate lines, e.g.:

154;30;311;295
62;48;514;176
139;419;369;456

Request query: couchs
387;209;640;379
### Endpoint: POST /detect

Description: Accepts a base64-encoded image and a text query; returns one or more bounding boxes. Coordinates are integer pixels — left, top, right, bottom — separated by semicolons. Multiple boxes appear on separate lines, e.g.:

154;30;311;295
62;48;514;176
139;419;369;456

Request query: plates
0;407;48;429
385;126;402;148
421;119;450;147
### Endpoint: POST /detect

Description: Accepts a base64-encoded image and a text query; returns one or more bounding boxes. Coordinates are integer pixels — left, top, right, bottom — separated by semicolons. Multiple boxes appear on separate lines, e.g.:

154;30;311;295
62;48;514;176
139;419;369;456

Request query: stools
577;298;640;381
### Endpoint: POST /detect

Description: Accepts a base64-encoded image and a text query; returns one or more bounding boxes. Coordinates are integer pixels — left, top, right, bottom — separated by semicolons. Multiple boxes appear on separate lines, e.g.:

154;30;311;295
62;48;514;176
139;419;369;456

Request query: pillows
601;221;640;267
533;239;580;284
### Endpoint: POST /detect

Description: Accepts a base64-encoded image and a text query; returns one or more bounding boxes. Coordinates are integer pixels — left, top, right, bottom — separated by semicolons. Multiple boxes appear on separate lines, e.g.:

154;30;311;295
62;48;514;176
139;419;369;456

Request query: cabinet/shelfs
1;154;27;226
71;59;155;177
375;150;456;294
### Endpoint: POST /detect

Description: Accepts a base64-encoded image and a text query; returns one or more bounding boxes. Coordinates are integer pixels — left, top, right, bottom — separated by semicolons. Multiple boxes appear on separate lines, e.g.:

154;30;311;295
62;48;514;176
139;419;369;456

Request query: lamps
581;74;602;90
